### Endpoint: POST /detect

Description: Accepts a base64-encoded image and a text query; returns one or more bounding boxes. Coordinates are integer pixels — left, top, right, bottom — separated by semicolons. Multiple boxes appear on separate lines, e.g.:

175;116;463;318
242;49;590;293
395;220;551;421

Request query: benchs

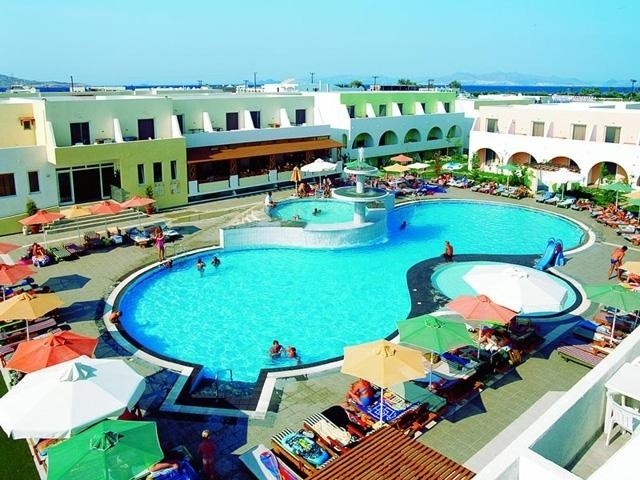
0;314;65;357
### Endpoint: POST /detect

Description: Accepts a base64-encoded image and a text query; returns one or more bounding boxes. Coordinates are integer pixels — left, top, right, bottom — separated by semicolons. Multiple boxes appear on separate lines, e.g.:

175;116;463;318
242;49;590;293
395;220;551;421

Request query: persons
606;245;627;281
285;346;302;365
147;452;178;472
399;221;407;229
154;227;166;262
211;256;221;269
594;203;640;247
295;172;536;200
265;191;277;208
344;374;374;406
269;340;284;364
164;259;174;269
198;258;207;275
443;240;453;257
198;429;218;480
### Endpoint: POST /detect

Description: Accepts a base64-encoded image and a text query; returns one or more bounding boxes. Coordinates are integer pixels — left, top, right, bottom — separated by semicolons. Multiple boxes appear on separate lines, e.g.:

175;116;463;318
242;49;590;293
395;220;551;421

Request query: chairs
366;176;533;202
536;187;640;244
270;386;436;476
25;219;181;269
591;301;639;356
392;318;542;424
309;175;357;200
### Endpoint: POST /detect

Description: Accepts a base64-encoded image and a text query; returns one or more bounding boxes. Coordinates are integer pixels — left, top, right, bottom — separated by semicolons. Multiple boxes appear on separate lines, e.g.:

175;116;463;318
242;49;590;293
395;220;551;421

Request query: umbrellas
443;162;463;179
339;339;437;427
0;242;165;479
121;195;155;233
289;154;430;189
394;313;476;391
443;294;520;360
17;210;65;251
87;201;122;237
59;203;92;247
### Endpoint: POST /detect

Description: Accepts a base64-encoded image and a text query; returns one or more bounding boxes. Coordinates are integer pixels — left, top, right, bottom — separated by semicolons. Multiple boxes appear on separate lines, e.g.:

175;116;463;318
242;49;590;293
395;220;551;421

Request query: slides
535;238;563;270
